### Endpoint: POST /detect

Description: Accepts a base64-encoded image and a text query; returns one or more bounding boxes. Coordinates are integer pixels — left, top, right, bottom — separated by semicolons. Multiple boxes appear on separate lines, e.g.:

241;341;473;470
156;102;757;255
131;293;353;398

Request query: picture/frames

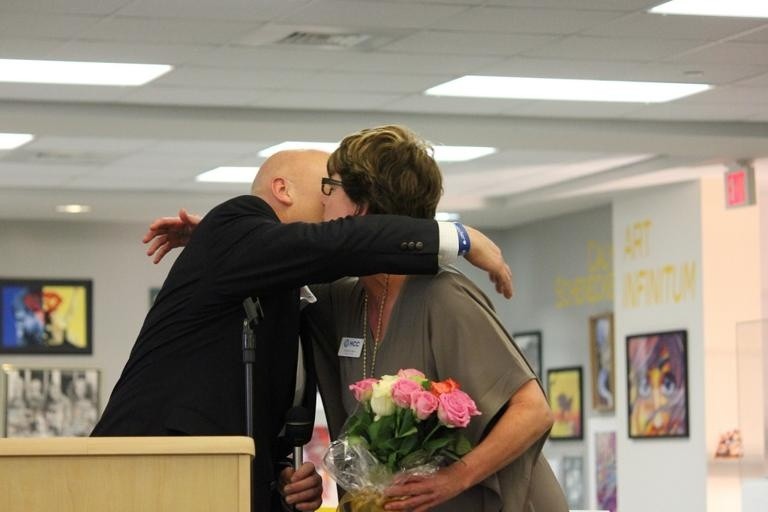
1;279;101;437
514;313;687;511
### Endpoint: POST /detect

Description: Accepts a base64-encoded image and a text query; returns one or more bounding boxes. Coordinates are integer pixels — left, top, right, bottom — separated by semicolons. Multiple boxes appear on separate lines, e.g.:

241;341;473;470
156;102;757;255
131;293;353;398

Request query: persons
88;149;513;512
143;125;570;512
628;333;686;436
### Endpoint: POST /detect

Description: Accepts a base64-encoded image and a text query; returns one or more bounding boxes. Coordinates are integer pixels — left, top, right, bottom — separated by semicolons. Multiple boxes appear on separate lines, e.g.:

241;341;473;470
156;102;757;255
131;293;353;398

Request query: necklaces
362;273;390;380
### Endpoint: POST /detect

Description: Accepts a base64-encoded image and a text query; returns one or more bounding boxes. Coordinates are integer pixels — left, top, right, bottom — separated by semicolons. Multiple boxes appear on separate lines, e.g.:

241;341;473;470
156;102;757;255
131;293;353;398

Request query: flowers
342;367;482;485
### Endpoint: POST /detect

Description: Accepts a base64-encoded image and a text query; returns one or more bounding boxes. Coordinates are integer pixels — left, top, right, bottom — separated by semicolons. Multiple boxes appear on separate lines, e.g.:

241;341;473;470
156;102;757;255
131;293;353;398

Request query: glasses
321;177;344;196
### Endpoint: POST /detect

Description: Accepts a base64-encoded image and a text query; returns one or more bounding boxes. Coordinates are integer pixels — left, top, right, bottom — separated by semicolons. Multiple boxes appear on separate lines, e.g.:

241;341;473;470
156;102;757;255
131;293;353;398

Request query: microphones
284;406;315;512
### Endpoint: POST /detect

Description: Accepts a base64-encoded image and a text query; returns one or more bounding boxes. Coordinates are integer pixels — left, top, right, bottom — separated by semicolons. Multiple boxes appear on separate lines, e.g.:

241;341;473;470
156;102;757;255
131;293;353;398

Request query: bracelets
454;222;470;257
